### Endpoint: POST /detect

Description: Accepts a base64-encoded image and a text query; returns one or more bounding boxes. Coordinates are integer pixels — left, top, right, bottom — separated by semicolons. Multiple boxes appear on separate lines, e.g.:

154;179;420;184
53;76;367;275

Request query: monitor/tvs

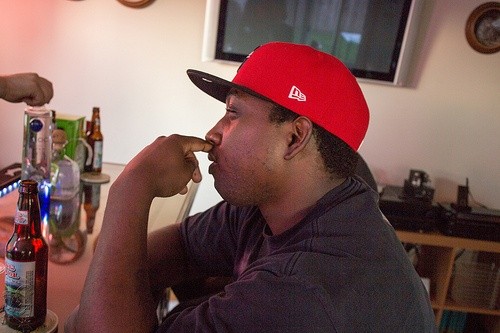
202;0;424;88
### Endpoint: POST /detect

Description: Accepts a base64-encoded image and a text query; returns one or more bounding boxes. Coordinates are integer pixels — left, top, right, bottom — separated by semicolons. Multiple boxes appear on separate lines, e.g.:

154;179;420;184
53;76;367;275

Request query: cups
408;169;426;188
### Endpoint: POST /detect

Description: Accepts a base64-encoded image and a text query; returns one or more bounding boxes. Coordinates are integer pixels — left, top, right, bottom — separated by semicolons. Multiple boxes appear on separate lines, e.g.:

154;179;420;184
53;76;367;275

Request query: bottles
21;101;50;194
84;107;103;173
82;183;100;233
4;179;49;332
49;109;57;160
47;130;80;200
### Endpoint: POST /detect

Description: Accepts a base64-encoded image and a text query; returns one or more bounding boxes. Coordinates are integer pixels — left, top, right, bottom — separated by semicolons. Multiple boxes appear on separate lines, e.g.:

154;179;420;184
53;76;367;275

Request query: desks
1;160;199;333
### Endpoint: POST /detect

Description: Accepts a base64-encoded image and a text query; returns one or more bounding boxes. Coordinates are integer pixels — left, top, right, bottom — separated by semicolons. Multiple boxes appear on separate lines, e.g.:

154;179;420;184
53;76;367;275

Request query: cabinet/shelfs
394;227;500;333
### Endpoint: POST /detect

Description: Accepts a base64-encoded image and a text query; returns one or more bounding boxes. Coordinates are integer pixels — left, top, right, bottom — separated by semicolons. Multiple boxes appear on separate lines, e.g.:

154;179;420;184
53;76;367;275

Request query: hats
187;42;370;153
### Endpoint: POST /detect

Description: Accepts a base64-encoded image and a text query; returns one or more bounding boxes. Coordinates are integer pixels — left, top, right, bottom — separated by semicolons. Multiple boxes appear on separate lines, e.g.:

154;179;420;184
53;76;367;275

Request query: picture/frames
466;2;500;54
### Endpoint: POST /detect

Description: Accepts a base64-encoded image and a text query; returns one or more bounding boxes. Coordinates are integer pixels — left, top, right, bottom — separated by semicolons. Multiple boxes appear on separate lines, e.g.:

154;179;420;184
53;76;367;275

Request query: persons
0;71;54;107
63;43;439;333
